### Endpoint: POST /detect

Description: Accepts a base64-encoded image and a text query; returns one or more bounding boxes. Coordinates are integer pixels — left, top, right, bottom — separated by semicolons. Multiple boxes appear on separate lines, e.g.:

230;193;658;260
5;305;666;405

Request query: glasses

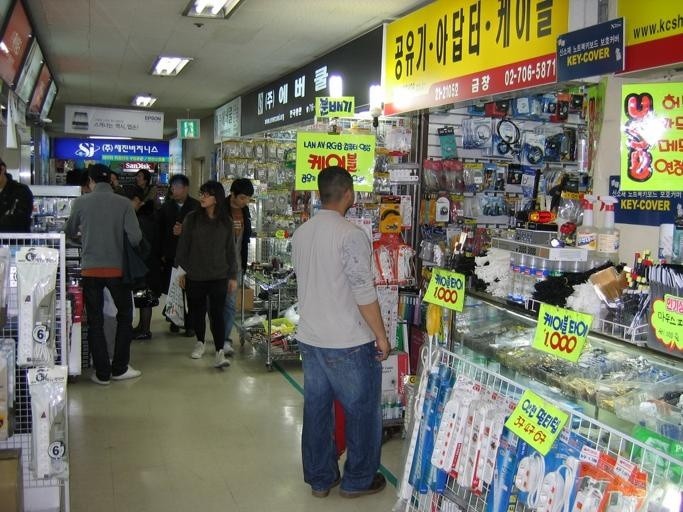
198;189;215;198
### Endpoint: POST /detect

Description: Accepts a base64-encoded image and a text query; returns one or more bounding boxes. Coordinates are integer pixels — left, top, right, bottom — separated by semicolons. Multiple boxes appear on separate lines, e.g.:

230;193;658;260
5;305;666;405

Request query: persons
291;167;391;499
0;160;33;434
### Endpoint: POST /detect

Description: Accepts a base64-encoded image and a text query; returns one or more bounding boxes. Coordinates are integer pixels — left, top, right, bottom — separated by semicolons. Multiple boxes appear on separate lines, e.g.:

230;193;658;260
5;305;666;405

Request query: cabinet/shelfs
239;265;300;372
431;287;682;512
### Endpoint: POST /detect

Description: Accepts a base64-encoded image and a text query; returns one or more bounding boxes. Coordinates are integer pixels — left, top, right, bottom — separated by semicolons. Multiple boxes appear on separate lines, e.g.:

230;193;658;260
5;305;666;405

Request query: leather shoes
311;470;341;498
339;473;386;499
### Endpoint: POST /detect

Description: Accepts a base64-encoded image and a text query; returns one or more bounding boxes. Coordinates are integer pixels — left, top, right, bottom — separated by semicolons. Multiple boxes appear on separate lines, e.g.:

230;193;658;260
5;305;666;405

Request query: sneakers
214;349;229;367
223;341;233;355
191;341;206;359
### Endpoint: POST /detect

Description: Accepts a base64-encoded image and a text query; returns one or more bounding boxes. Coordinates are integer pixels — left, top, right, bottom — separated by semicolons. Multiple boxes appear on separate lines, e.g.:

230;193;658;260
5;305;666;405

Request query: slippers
89;369;112;385
111;364;142;380
